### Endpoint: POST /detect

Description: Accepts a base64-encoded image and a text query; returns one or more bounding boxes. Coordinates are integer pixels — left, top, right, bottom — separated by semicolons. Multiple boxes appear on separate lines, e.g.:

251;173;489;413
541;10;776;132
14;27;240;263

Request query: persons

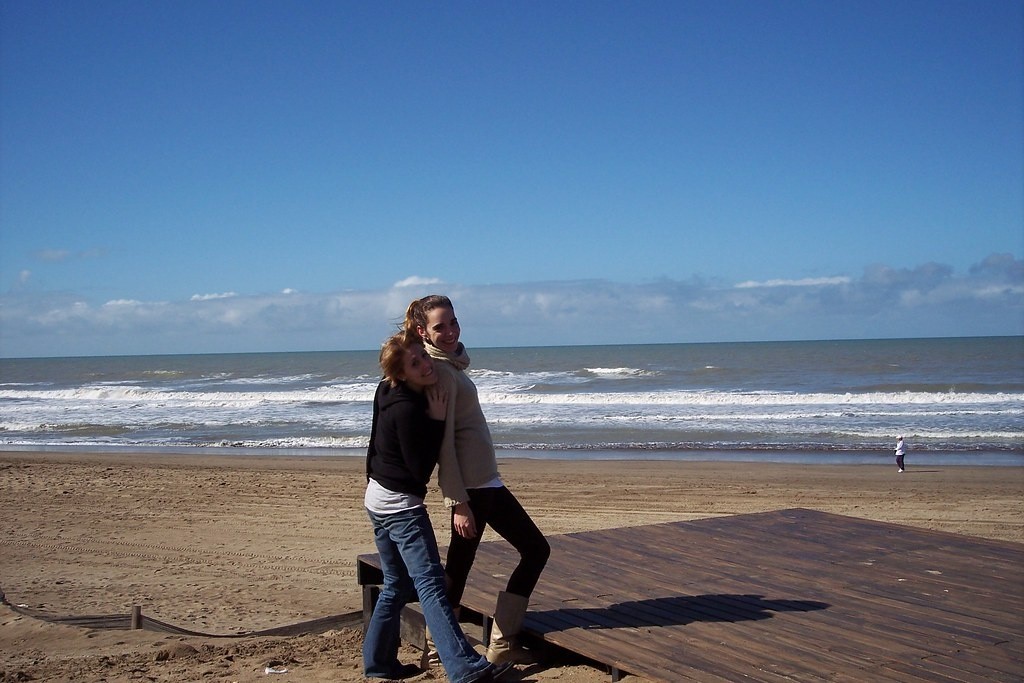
391;295;550;665
362;328;516;683
894;435;905;473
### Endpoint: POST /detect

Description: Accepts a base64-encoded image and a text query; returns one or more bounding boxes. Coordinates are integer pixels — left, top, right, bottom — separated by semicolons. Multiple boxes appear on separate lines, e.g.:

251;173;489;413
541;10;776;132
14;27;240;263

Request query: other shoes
382;660;419;679
470;659;514;683
898;468;904;473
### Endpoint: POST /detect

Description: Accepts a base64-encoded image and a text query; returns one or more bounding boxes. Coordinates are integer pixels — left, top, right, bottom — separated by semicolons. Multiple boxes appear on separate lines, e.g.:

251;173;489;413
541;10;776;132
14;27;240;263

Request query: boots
420;606;474;669
487;591;536;666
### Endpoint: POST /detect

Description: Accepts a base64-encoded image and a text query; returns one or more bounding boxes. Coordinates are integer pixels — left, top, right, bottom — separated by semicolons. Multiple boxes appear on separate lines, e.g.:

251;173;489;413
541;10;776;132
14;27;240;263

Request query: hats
896;436;902;439
